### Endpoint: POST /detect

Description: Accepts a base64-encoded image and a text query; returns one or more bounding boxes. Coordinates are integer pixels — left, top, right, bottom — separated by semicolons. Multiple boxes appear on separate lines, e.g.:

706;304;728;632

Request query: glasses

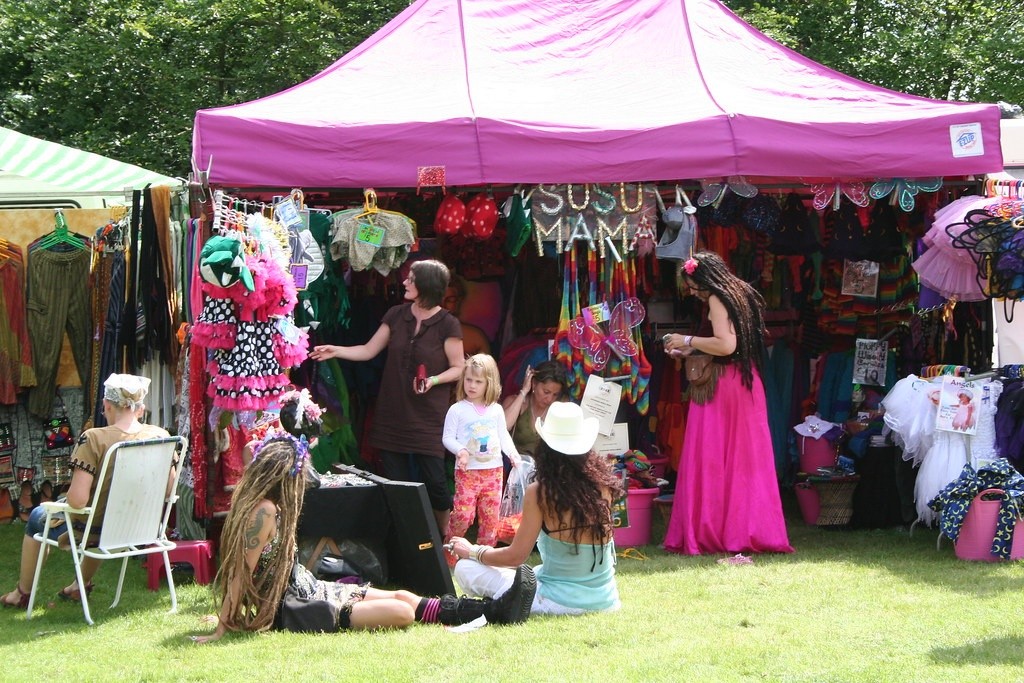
406;277;415;285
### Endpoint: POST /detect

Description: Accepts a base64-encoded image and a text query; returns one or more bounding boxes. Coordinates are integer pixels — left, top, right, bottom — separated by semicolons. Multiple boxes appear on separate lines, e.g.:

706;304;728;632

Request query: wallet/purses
416;364;427;394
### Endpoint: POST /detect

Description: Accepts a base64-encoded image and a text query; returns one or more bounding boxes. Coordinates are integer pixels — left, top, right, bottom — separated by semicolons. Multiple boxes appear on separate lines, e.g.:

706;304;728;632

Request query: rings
192;636;197;640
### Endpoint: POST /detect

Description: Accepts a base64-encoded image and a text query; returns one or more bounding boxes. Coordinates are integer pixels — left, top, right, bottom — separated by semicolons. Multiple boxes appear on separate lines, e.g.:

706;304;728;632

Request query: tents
195;0;1003;205
0;125;190;489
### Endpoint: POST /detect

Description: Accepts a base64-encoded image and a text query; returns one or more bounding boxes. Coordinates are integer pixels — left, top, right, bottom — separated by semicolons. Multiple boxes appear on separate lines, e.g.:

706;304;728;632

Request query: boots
437;563;536;625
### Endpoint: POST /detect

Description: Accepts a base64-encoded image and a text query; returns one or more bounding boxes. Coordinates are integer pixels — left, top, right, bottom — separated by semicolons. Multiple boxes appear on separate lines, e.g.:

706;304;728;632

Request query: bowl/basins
942;489;1024;561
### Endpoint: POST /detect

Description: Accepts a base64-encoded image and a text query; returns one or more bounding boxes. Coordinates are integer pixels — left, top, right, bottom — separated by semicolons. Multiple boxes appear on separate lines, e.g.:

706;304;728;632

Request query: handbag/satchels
496;455;538;545
685;355;712;384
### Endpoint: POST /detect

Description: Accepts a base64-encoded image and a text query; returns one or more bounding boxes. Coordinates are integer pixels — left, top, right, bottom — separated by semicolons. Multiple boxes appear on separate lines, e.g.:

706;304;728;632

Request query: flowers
684;257;699;276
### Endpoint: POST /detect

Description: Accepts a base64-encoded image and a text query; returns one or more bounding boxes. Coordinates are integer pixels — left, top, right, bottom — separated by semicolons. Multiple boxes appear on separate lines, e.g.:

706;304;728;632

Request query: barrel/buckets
795;482;820;525
612;487;659;547
797;434;835;474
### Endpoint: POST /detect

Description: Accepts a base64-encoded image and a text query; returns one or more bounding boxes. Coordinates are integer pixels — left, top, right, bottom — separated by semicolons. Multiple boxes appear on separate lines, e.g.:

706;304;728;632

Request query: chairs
25;435;188;626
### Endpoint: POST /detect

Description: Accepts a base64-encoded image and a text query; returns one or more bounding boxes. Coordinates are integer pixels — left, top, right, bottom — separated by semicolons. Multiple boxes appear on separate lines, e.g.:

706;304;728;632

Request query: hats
535;401;600;455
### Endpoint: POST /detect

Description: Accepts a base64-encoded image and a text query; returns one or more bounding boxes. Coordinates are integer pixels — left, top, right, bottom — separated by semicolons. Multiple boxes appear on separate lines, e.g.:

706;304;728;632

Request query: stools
143;537;217;592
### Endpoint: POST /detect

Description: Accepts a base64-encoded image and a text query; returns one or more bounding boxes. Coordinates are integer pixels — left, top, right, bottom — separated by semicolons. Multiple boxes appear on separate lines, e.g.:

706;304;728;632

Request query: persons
442;353;521;568
309;259;464;534
666;250;794;554
188;433;535;643
0;373;179;608
500;361;569;477
448;402;624;616
440;270;491;359
948;390;975;430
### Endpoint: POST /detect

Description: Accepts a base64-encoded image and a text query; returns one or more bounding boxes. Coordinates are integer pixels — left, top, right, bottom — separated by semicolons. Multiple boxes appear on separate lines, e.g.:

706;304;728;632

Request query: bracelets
520;389;526;402
468;544;494;565
685;336;694;347
432;376;438;385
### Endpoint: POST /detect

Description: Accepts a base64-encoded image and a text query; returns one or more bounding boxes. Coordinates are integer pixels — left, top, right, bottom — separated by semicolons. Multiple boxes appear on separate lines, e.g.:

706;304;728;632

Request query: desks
824;446;936;532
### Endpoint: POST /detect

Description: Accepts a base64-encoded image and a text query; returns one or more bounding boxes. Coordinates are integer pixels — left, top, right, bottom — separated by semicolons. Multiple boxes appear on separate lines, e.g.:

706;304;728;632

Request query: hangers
919;364;1024;384
984;178;1024;230
224;187;378;255
0;212;91;261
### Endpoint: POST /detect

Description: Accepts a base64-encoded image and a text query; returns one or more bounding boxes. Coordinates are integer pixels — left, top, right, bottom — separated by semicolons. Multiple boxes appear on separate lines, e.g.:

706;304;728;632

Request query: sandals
16;480;33;521
0;584;34;609
39;480;52;506
58;576;91;601
